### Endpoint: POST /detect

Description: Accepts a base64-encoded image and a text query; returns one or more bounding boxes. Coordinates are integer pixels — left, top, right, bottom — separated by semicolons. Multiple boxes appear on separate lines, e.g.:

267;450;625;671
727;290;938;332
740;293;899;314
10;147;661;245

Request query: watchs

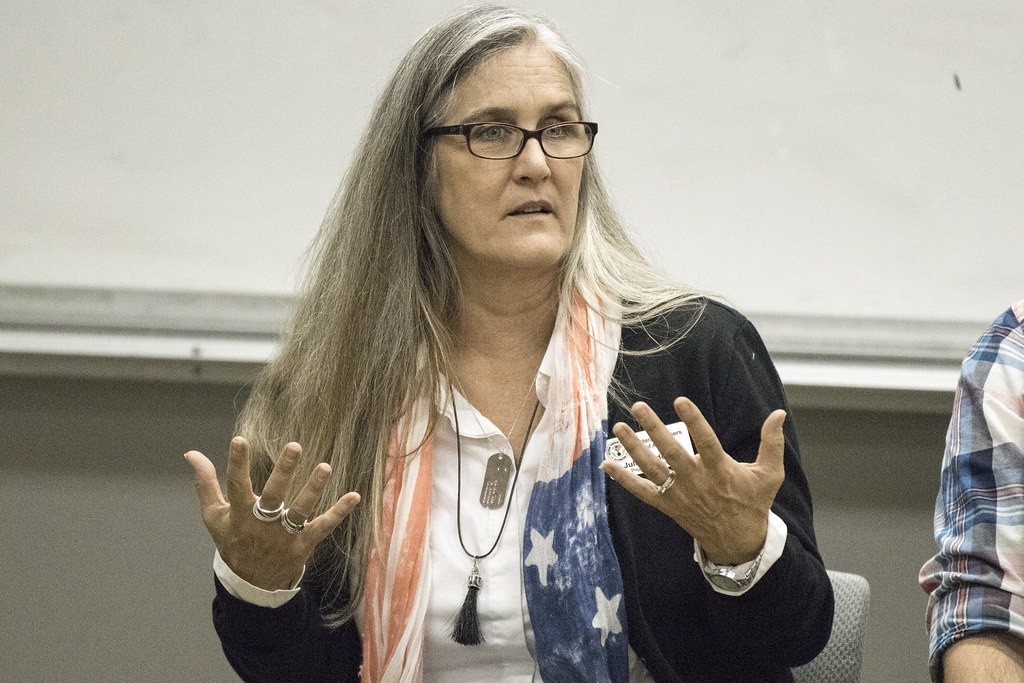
700;545;764;592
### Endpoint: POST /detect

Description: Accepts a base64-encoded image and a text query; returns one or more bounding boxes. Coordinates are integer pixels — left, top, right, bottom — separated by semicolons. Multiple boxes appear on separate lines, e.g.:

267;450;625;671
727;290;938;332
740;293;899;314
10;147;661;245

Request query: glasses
428;120;599;160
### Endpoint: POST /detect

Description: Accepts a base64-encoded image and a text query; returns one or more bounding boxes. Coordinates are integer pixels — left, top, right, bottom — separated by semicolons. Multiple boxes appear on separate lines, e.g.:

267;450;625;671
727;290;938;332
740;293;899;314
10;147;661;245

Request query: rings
253;498;306;535
658;474;675;495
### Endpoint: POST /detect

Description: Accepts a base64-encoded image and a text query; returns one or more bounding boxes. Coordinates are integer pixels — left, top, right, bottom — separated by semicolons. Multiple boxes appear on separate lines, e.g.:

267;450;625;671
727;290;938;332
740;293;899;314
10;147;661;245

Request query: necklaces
442;348;543;644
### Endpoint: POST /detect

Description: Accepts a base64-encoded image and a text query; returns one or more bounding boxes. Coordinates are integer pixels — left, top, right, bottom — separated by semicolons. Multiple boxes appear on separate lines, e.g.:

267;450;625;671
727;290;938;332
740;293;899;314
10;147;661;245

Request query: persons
918;300;1024;683
183;6;833;683
